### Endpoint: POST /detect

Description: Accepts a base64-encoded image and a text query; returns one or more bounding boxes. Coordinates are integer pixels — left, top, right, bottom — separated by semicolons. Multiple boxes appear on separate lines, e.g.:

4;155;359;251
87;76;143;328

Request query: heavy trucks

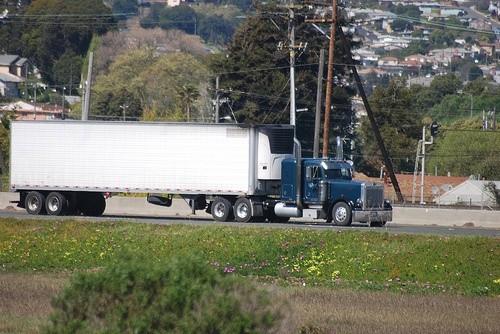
9;120;391;228
